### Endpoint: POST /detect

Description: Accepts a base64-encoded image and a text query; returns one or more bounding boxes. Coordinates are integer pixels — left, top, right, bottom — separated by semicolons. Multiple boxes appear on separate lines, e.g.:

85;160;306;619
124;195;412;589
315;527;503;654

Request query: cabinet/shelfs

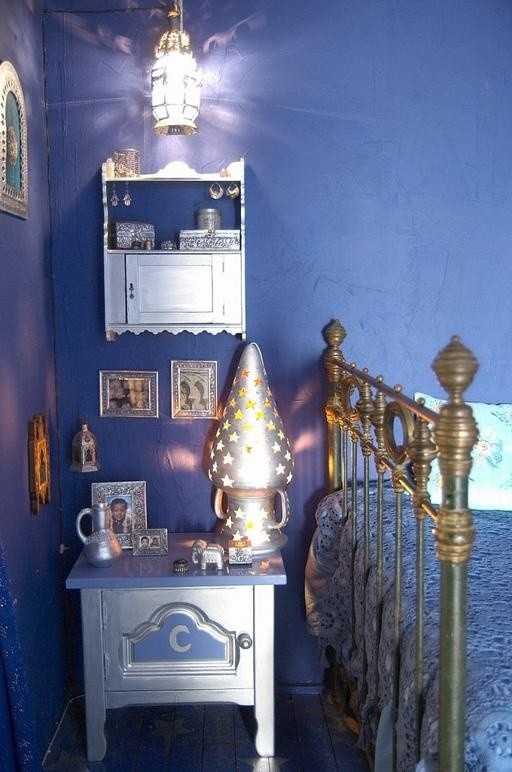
100;148;247;342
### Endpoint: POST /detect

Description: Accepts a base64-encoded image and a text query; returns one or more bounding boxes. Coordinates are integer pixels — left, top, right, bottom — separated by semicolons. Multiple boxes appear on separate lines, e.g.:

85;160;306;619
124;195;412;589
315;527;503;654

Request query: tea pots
74;497;122;567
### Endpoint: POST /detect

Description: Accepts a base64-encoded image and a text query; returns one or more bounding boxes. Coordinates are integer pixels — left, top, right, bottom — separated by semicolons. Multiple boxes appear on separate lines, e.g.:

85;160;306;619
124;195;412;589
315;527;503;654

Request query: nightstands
64;532;288;761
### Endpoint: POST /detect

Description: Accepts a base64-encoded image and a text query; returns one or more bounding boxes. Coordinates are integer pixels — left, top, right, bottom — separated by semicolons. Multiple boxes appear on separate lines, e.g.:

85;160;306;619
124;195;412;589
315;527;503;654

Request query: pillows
407;390;512;512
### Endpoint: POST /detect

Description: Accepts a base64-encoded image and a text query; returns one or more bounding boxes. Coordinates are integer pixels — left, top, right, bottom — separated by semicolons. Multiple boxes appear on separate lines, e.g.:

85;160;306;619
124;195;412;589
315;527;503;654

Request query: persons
108;499;133;534
109;379;148;410
151;537;160;547
180;381;192;410
139;536;150;550
191;381;206;411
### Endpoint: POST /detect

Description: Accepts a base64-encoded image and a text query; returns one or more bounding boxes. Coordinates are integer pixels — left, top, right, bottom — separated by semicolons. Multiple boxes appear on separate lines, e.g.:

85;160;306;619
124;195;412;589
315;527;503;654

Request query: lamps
208;338;296;560
147;0;204;134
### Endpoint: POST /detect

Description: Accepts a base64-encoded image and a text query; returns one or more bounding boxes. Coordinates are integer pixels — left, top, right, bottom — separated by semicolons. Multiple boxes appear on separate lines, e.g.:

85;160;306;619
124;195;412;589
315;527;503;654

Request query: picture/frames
97;369;158;419
26;412;53;517
132;528;169;556
1;59;29;222
90;479;148;550
169;359;219;421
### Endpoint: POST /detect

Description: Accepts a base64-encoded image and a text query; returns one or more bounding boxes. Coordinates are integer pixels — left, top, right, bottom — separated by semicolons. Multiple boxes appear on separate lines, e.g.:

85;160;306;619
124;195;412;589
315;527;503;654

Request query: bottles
144;236;152;251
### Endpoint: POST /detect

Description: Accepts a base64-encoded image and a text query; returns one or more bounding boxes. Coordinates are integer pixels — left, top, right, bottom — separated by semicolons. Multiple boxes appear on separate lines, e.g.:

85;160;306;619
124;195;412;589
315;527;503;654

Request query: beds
306;315;512;772
